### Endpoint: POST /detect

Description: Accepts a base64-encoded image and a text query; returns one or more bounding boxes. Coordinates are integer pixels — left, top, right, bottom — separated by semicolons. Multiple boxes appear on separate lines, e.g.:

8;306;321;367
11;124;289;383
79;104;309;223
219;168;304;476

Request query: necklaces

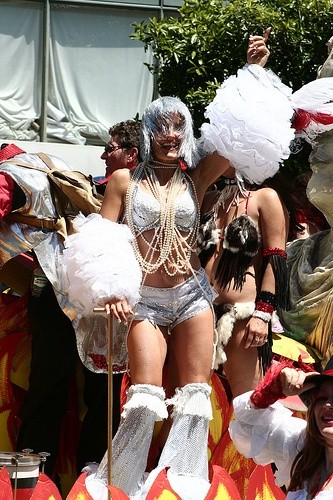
126;158;219;374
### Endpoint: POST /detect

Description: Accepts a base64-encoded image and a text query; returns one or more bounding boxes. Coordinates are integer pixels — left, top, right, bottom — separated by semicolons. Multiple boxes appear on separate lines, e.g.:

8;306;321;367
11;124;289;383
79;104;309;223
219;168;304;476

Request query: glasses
104;144;120;153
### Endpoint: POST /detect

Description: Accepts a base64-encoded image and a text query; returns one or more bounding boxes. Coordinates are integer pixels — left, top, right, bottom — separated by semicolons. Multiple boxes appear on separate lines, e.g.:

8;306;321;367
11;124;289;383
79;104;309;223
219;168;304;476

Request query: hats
293;355;333;405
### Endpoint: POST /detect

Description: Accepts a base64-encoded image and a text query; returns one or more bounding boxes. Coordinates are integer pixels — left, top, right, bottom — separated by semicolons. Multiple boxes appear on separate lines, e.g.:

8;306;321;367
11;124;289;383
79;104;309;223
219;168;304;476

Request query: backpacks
1;151;106;250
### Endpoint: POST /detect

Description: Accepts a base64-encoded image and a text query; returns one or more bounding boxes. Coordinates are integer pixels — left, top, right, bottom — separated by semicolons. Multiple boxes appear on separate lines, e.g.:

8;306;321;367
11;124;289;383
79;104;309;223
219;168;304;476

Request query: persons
200;165;333;500
57;26;293;497
271;30;333;370
0;121;147;476
